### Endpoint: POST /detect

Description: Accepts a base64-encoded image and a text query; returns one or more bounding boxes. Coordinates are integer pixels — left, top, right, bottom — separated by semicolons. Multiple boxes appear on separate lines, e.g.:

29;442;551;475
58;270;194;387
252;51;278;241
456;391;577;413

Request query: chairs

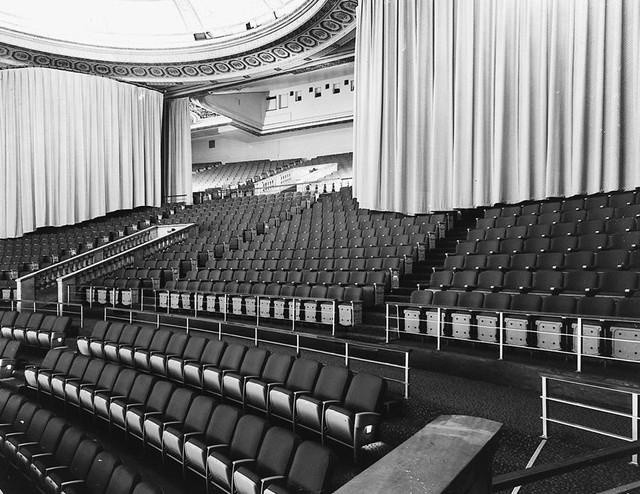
0;153;640;494
